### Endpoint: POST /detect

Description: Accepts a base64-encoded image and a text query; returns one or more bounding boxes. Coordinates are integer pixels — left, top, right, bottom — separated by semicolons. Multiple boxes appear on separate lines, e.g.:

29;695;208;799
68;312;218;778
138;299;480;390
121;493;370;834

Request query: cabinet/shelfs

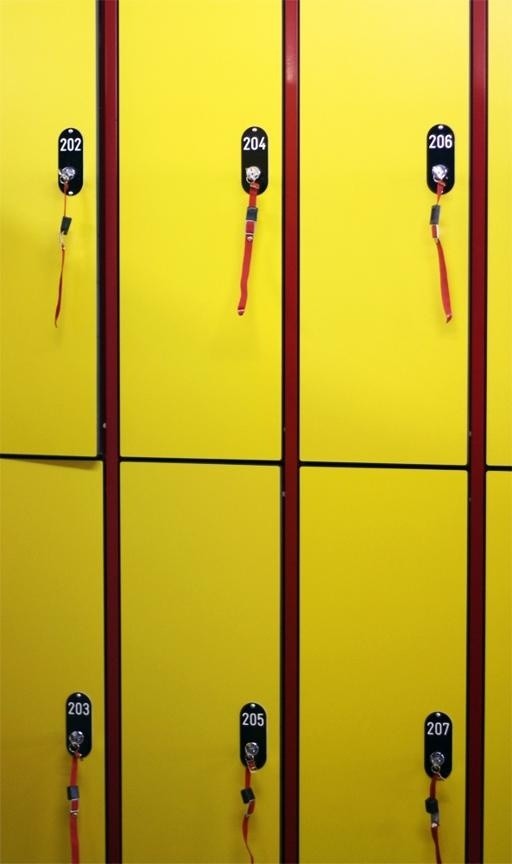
106;466;280;857
2;455;119;859
98;2;300;466
475;478;511;859
293;468;477;857
282;2;480;471
469;2;512;471
0;2;108;455
